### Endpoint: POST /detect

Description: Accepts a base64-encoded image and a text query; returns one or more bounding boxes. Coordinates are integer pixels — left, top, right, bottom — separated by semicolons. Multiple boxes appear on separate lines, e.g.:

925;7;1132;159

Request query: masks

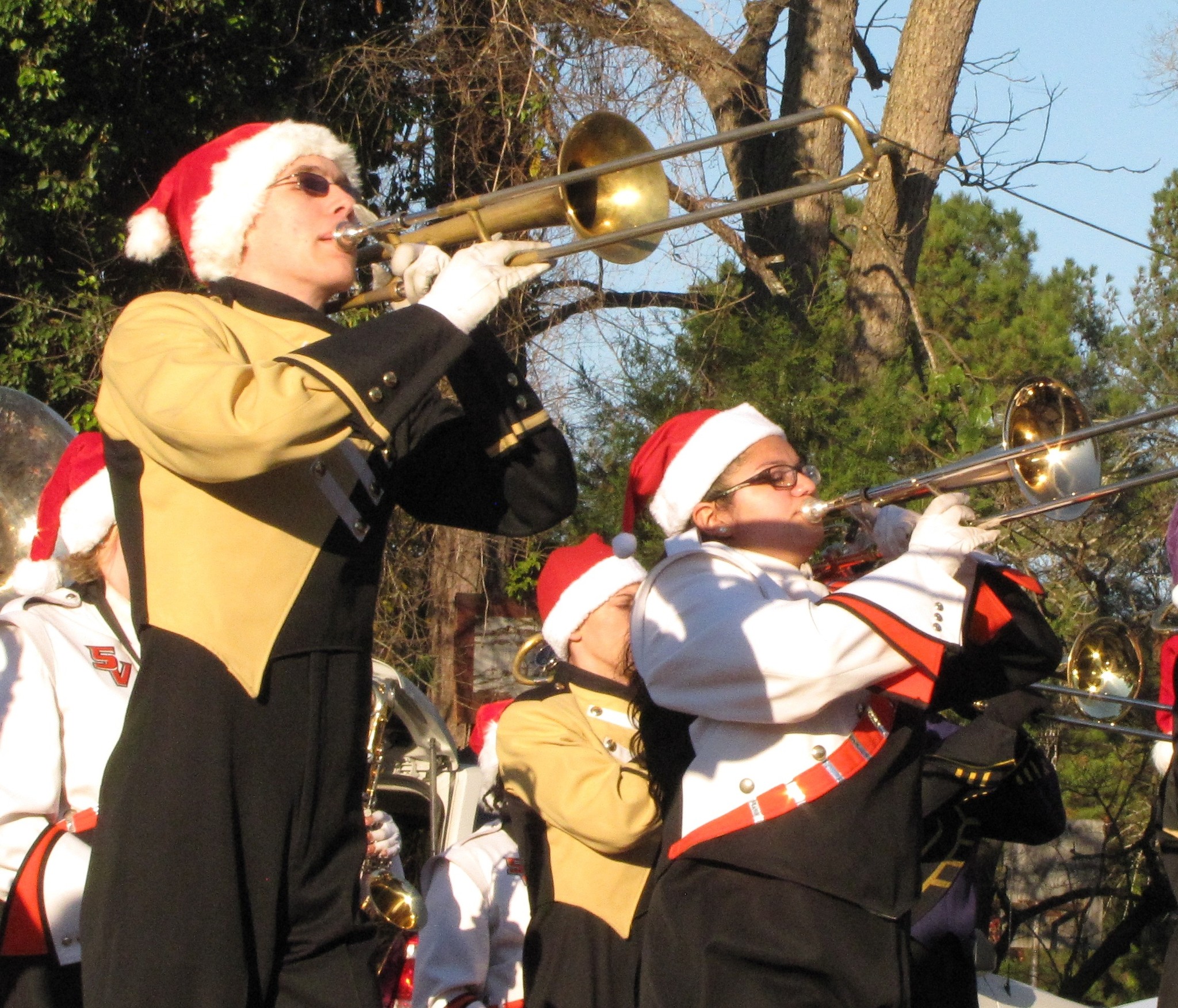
536;533;648;665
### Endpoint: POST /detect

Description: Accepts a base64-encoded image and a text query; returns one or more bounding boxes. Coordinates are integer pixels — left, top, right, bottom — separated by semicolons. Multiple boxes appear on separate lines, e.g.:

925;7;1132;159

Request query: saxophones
353;678;428;975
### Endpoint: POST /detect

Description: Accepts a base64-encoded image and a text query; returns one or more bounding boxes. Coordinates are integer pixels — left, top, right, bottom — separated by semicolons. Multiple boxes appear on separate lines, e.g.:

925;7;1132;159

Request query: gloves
874;505;922;561
417;241;557;336
904;491;1002;561
370;241;451;308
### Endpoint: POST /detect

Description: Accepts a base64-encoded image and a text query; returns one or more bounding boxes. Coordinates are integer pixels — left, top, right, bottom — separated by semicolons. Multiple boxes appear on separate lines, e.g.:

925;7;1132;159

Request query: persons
499;535;661;1008
79;121;579;1008
412;700;531;1008
1157;503;1178;1008
908;686;1066;1008
613;402;1062;1008
0;432;406;1008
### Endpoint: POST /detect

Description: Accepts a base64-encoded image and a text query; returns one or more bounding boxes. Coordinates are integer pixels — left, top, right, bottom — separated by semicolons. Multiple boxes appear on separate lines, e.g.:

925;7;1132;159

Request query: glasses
269;172;366;204
708;464;821;503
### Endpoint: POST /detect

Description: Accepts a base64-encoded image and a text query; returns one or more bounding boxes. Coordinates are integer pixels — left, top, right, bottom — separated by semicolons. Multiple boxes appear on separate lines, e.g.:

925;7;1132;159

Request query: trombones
327;104;881;309
799;382;1175;571
1027;620;1174;745
511;633;559;686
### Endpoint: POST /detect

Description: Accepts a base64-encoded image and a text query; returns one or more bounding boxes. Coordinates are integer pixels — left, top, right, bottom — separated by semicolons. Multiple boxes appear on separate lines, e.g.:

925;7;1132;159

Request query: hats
125;123;363;281
469;699;523;785
612;403;787;560
12;431;117;592
